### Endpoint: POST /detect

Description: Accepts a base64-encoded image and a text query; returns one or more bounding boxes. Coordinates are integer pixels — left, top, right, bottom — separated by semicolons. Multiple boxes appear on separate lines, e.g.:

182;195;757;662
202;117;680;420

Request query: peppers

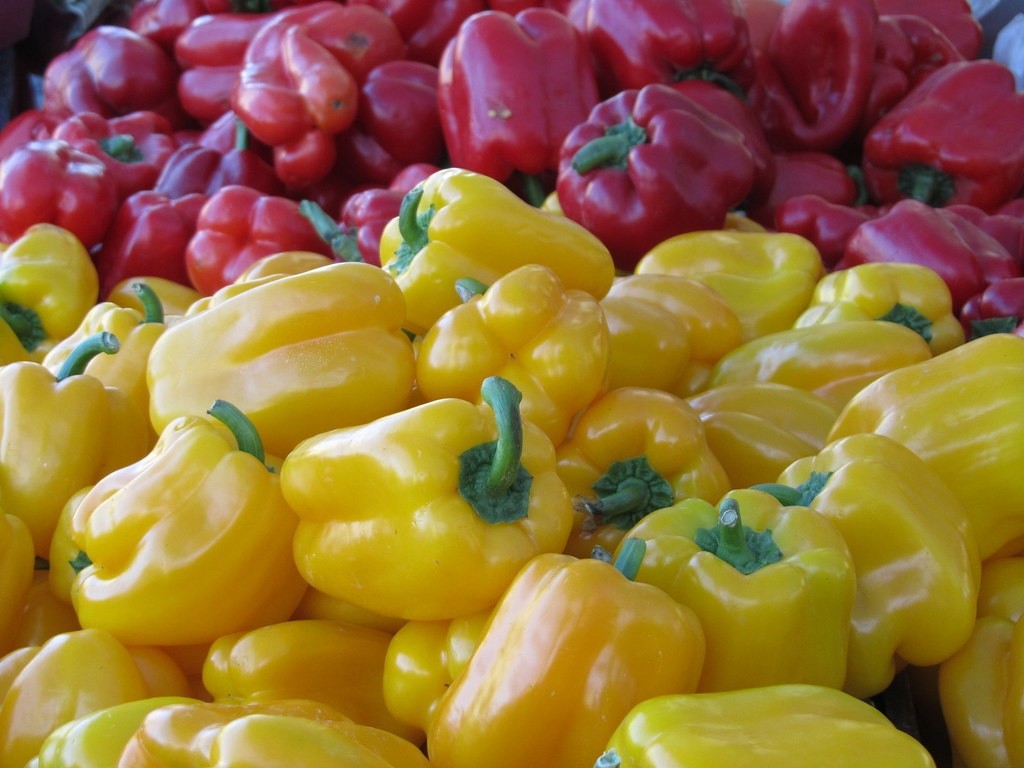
0;0;1024;768
